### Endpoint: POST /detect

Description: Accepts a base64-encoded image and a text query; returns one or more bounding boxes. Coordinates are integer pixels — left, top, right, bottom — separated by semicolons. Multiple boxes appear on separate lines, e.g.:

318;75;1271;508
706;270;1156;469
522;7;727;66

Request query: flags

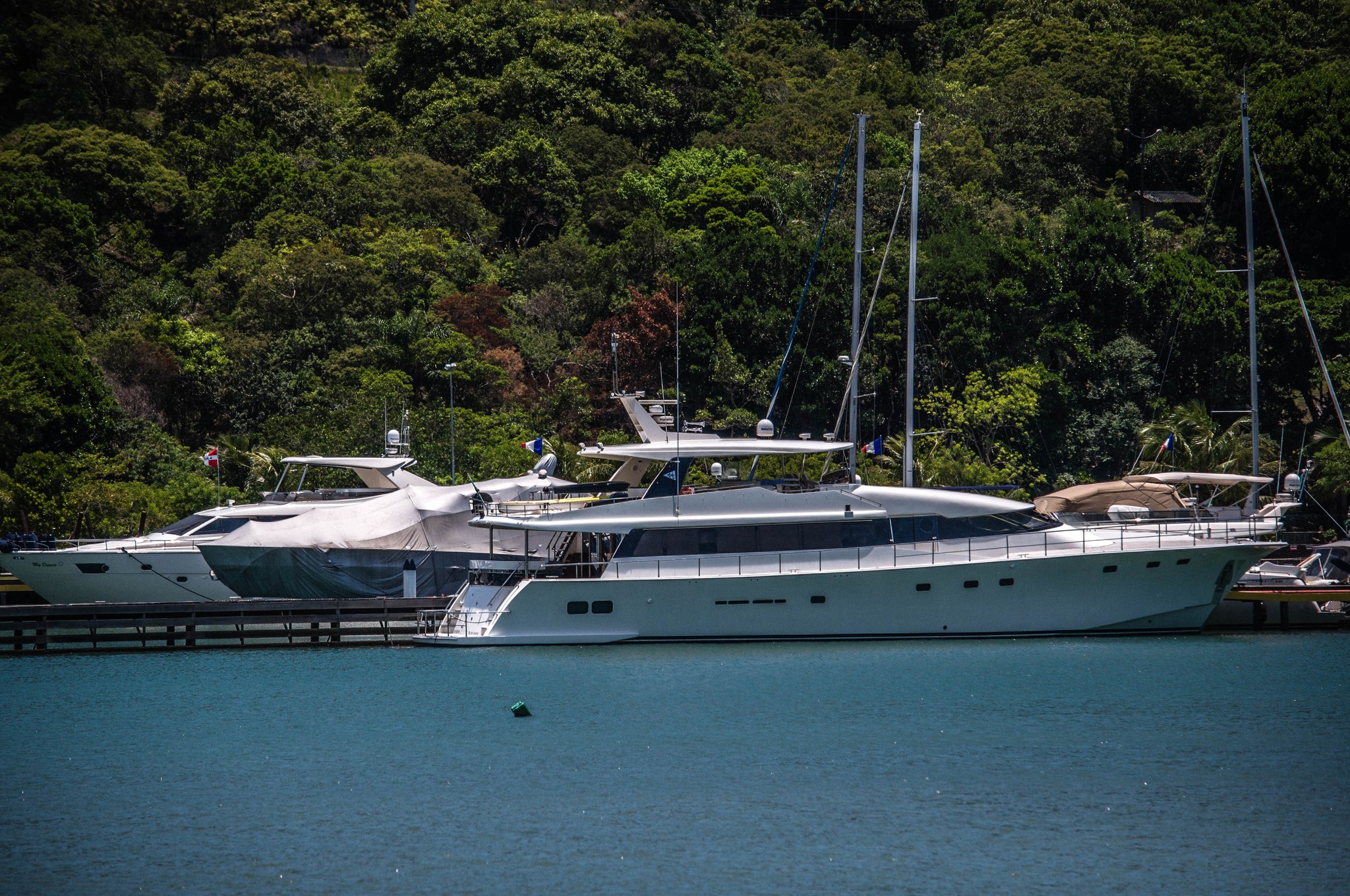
519;437;542;456
862;437;882;456
1160;432;1174;453
204;448;218;467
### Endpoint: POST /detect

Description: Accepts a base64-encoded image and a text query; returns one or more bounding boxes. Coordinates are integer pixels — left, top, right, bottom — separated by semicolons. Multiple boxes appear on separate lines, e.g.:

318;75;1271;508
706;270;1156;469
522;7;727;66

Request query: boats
0;100;1350;642
